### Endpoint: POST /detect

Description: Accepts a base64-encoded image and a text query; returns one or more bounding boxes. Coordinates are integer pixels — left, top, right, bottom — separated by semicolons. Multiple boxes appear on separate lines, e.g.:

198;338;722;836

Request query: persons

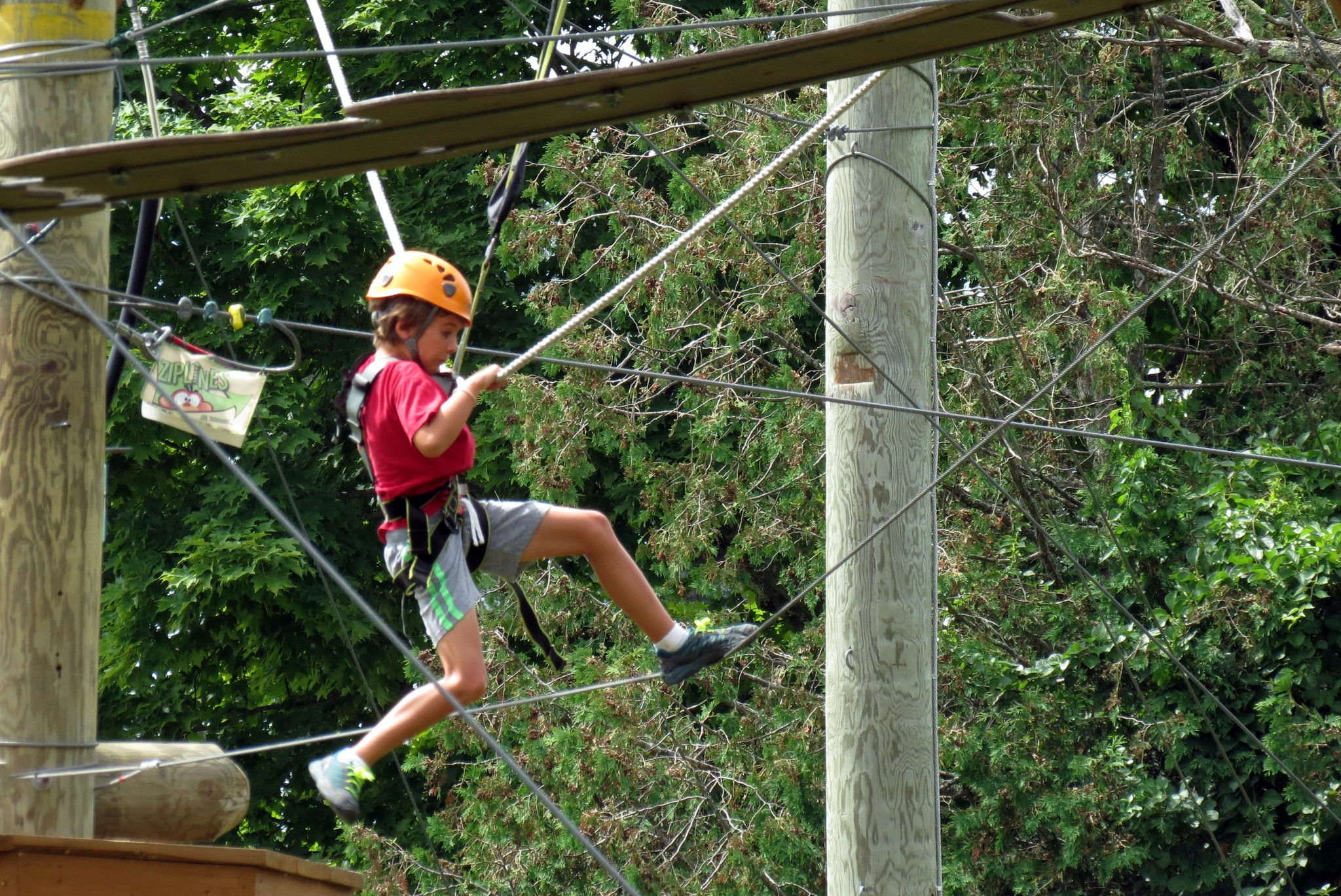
306;252;757;826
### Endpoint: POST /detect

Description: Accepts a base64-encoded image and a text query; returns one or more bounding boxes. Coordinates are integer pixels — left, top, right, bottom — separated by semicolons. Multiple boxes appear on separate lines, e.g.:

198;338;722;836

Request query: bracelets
452;388;477;407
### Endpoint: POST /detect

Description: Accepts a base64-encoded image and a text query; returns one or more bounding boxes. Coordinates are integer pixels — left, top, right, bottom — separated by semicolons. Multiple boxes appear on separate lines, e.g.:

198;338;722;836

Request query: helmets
365;251;472;326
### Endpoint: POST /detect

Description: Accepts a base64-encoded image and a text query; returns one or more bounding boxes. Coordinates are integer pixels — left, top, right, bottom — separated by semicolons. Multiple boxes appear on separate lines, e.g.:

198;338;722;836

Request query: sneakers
309;748;375;824
649;618;761;685
